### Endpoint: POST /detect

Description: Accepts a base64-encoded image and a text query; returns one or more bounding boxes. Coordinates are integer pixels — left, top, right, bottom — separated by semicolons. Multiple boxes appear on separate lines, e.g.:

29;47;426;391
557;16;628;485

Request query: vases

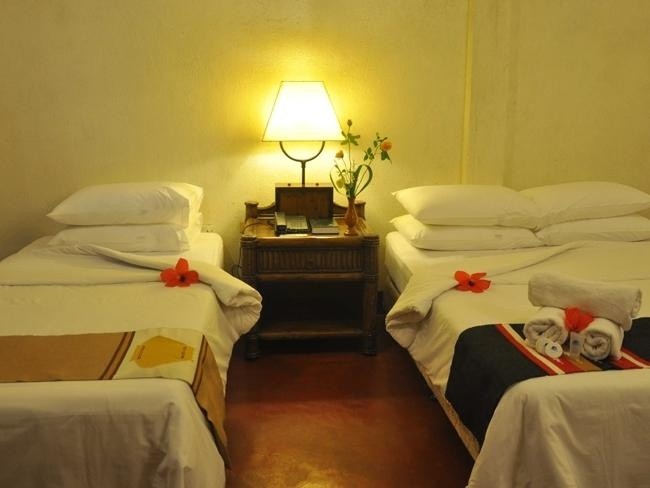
347;198;358;236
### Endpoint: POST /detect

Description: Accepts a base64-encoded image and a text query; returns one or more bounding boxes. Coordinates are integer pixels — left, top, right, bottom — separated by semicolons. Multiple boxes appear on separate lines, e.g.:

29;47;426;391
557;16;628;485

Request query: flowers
330;119;394;197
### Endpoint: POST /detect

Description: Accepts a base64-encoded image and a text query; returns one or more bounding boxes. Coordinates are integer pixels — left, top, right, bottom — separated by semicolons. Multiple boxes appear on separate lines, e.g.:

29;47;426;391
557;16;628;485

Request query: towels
528;270;642;331
523;307;568;348
579;318;624;361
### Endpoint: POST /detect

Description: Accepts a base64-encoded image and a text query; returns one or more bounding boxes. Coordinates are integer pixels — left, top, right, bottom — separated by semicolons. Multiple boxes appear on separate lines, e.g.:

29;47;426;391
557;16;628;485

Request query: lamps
261;81;345;217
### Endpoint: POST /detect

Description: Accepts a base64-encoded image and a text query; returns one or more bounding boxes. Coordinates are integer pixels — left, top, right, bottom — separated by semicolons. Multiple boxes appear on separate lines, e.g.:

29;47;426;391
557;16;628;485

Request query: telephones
274;211;309;233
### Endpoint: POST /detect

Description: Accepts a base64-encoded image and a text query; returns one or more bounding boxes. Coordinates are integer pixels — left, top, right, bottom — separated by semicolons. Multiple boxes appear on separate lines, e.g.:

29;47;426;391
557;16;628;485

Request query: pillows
393;186;546;229
46;183;202;225
520;181;650;230
390;214;545;250
49;226;201;253
537;214;650;246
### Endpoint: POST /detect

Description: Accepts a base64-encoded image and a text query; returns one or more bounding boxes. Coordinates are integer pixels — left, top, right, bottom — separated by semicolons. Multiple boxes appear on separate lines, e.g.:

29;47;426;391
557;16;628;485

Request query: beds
0;232;262;488
385;231;650;488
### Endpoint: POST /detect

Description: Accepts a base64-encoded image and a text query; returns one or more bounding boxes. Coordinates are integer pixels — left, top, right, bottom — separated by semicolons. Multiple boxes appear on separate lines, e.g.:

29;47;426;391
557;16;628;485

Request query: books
309;215;340;234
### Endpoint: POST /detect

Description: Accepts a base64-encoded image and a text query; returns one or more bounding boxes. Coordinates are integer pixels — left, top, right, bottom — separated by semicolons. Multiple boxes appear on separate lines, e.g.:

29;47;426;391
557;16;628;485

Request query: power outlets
207;229;214;233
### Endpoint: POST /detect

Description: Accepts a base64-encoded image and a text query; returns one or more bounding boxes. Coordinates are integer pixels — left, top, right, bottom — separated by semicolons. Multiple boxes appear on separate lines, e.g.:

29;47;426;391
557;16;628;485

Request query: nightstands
240;202;380;360
